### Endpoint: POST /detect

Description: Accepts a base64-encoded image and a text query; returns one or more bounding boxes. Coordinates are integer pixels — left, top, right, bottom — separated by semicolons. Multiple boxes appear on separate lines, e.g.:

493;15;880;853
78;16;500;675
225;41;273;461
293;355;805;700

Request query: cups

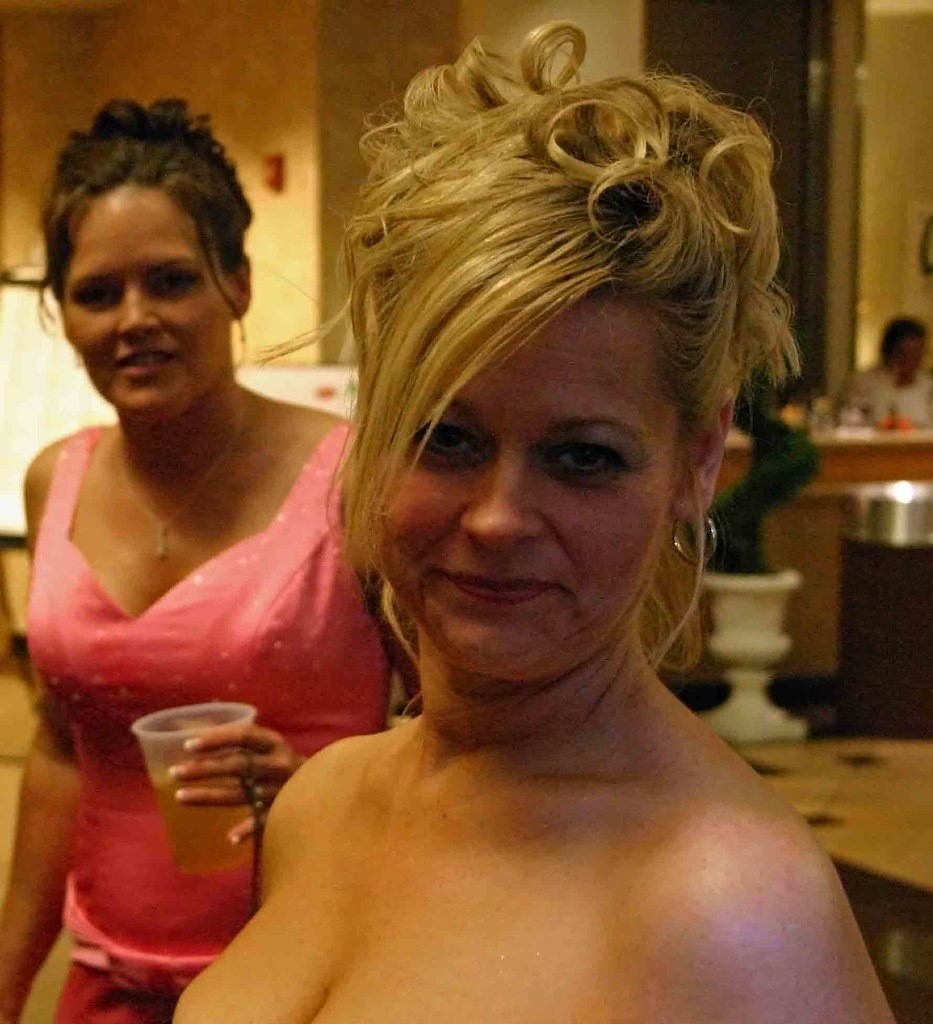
132;704;258;873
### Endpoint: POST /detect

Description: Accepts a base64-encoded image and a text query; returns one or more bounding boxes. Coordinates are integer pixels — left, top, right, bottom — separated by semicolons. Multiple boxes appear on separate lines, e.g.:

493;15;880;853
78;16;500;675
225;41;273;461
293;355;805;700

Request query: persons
843;312;932;429
0;93;424;1024
168;21;896;1024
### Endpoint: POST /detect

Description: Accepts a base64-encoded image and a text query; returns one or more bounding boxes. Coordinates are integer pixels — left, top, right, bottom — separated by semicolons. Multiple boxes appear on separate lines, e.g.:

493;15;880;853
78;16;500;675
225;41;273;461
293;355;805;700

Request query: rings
241;771;267;814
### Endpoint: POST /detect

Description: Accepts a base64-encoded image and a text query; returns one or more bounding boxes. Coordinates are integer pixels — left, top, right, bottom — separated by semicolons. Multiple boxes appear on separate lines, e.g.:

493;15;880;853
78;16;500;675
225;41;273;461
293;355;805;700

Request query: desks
766;434;933;672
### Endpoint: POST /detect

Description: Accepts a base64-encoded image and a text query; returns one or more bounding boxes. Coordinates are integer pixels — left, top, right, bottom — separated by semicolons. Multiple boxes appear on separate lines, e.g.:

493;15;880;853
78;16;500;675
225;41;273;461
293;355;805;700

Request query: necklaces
117;398;244;559
